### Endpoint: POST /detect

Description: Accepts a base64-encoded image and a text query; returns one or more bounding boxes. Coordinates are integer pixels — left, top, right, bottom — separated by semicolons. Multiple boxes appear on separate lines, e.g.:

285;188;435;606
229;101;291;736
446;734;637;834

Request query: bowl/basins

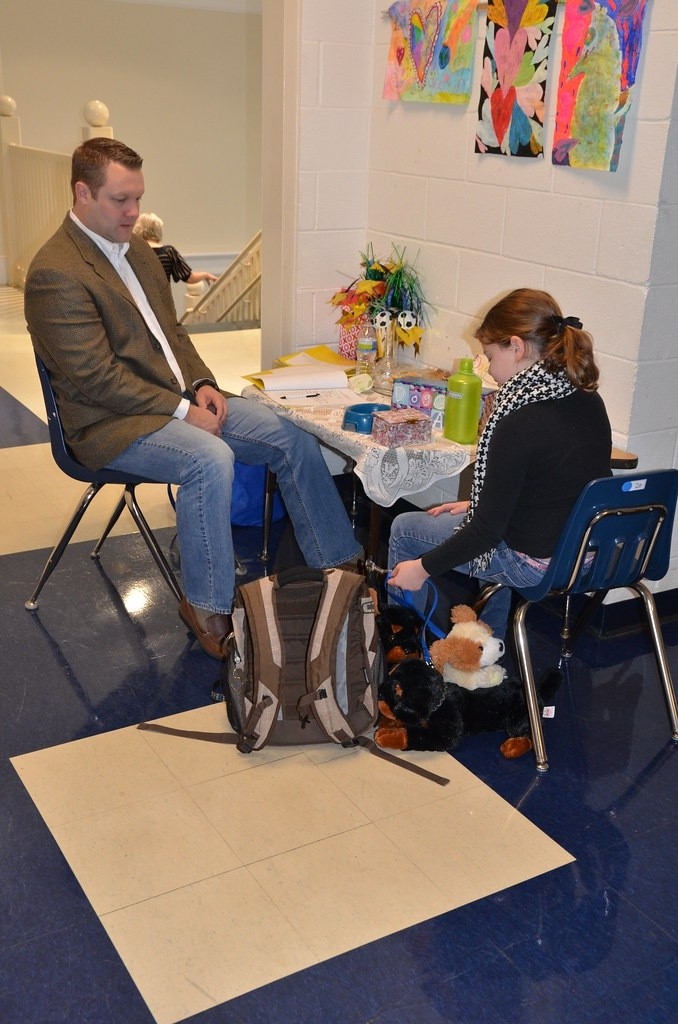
342;403;393;434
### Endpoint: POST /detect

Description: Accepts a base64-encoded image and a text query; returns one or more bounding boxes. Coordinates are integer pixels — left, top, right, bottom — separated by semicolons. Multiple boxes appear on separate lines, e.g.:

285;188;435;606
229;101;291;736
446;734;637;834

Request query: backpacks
221;567;386;752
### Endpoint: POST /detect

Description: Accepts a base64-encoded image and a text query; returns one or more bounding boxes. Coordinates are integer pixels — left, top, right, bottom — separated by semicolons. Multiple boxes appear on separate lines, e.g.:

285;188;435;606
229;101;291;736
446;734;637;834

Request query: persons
386;288;614;646
25;134;373;659
132;213;219;286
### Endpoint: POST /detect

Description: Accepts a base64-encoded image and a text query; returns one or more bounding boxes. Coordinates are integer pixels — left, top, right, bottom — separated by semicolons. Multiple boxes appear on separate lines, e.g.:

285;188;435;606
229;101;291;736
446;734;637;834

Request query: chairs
25;326;248;609
472;467;678;773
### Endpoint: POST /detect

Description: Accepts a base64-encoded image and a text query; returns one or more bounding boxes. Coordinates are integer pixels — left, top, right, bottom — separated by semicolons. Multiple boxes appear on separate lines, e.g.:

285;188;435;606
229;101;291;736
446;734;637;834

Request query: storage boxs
371;408;432;448
392;375;498;430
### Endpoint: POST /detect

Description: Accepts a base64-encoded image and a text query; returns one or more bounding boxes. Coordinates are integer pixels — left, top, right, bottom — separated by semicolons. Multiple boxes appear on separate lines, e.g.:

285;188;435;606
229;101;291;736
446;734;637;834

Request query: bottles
444;358;482;444
356;322;376;377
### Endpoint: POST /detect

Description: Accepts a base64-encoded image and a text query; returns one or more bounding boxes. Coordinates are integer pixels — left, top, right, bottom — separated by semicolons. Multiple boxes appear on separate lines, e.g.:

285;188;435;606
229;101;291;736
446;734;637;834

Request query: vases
373;325;400;374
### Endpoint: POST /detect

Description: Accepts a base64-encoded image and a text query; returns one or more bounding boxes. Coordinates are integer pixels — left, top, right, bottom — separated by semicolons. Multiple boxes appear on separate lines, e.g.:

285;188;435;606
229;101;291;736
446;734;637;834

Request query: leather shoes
178;596;233;661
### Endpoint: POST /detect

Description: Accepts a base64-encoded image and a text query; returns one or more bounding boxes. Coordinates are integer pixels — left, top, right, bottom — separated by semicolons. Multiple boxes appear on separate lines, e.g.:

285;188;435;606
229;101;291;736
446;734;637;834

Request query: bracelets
194;380;220;396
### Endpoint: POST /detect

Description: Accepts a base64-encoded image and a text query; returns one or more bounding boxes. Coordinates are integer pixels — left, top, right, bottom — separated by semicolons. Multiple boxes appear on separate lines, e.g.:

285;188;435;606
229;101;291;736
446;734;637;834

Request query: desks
243;385;640;562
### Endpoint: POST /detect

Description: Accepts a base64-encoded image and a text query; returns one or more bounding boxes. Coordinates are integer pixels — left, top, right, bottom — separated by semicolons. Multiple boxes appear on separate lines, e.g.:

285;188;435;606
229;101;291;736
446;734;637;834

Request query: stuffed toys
374;607;424;663
372;659;561;760
429;603;506;691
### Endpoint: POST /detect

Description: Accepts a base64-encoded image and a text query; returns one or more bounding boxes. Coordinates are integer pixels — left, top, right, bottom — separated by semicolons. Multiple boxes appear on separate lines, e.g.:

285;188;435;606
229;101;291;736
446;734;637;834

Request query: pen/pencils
280;393;320;400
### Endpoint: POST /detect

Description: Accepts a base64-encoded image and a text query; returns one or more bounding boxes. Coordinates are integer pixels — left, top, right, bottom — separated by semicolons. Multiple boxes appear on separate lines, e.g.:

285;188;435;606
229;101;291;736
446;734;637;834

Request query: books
252;363;368;410
